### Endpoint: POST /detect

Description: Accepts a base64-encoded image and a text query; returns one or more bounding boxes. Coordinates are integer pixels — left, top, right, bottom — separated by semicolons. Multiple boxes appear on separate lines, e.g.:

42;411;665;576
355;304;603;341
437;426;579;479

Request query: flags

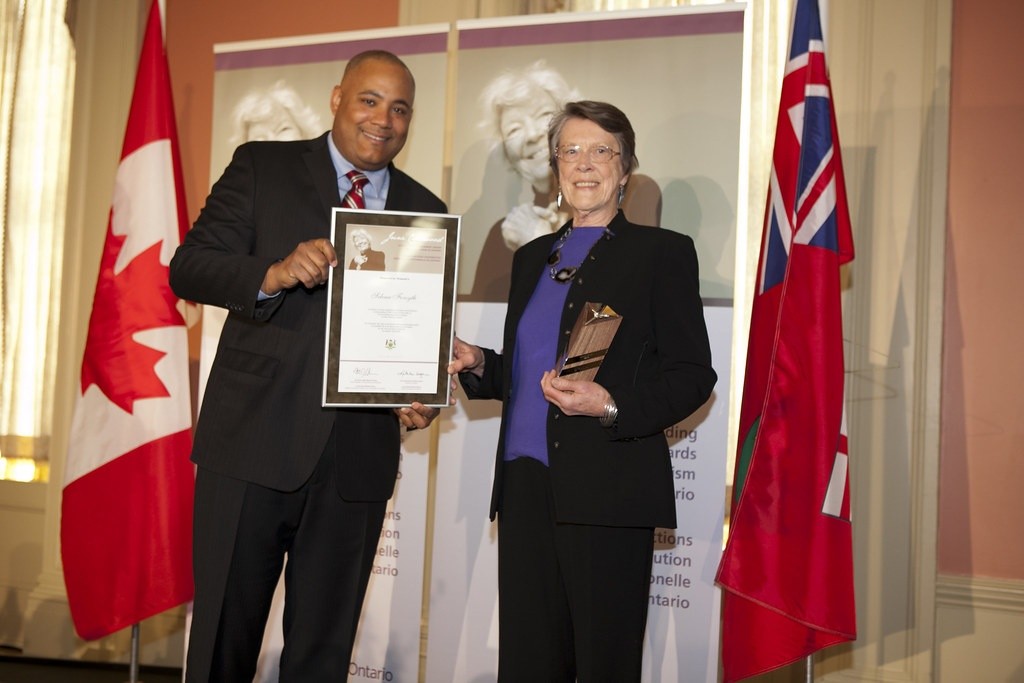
57;1;197;645
707;0;863;681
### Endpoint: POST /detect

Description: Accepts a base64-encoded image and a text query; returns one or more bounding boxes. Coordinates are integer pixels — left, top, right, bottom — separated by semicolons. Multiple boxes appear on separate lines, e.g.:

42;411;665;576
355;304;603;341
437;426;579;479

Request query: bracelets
599;395;618;427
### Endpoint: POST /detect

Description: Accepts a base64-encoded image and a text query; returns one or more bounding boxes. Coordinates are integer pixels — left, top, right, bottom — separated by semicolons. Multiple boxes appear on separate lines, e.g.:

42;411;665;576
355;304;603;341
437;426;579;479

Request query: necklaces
543;225;604;283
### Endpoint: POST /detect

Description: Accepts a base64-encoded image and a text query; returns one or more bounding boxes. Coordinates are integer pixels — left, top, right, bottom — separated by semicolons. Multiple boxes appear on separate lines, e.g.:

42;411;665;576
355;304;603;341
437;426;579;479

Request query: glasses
552;143;621;162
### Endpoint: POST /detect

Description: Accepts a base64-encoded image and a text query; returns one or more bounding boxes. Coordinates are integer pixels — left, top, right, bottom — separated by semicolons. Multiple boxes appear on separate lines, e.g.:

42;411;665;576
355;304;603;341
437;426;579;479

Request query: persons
347;227;386;271
445;98;718;683
164;49;451;682
227;80;322;149
472;62;660;304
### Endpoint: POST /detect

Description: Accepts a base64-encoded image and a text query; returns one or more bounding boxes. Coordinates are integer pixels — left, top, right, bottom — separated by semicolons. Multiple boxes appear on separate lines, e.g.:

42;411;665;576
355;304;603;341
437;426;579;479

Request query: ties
342;171;370;209
